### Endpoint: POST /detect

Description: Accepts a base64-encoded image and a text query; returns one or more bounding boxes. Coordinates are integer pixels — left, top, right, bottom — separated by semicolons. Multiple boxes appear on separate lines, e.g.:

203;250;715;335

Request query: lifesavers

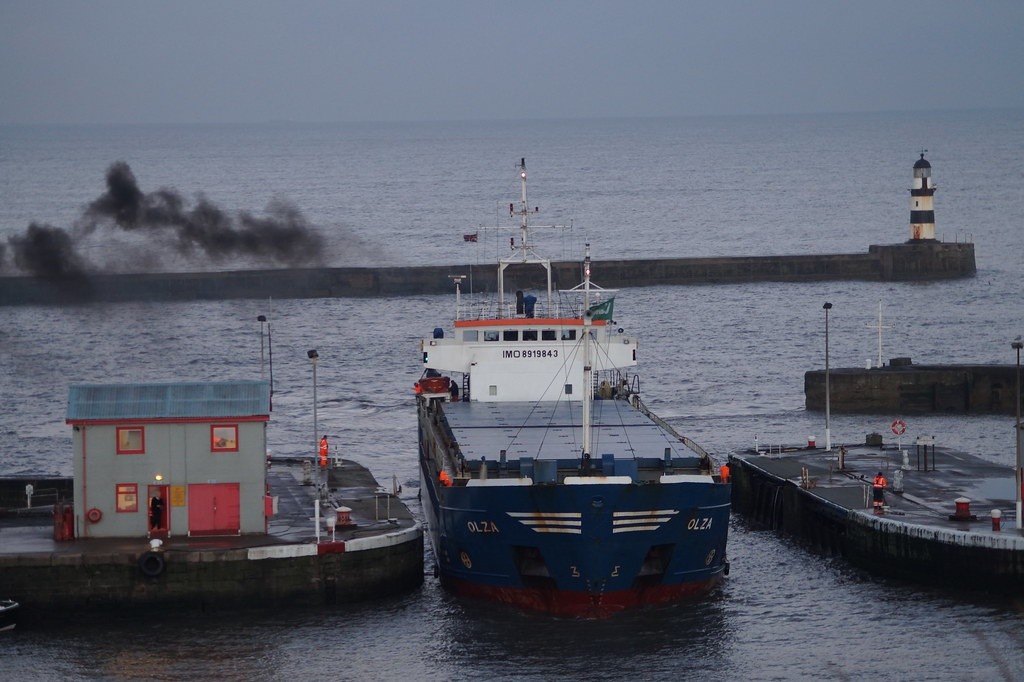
141;553;163;577
88;507;101;521
892;419;906;435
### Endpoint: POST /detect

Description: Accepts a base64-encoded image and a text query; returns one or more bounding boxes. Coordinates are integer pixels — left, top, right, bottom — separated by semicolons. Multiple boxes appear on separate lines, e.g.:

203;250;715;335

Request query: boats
413;157;732;626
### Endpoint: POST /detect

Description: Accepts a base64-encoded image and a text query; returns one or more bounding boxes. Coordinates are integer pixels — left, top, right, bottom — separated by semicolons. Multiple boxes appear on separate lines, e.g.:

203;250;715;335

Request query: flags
463;234;477;242
589;298;613;320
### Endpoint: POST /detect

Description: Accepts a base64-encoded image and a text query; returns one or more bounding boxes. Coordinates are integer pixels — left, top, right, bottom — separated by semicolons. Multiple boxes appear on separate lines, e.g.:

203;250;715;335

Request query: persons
151;497;161;529
873;471;886;514
720;463;731;483
449;380;459;402
320;435;328;471
414;383;420;393
